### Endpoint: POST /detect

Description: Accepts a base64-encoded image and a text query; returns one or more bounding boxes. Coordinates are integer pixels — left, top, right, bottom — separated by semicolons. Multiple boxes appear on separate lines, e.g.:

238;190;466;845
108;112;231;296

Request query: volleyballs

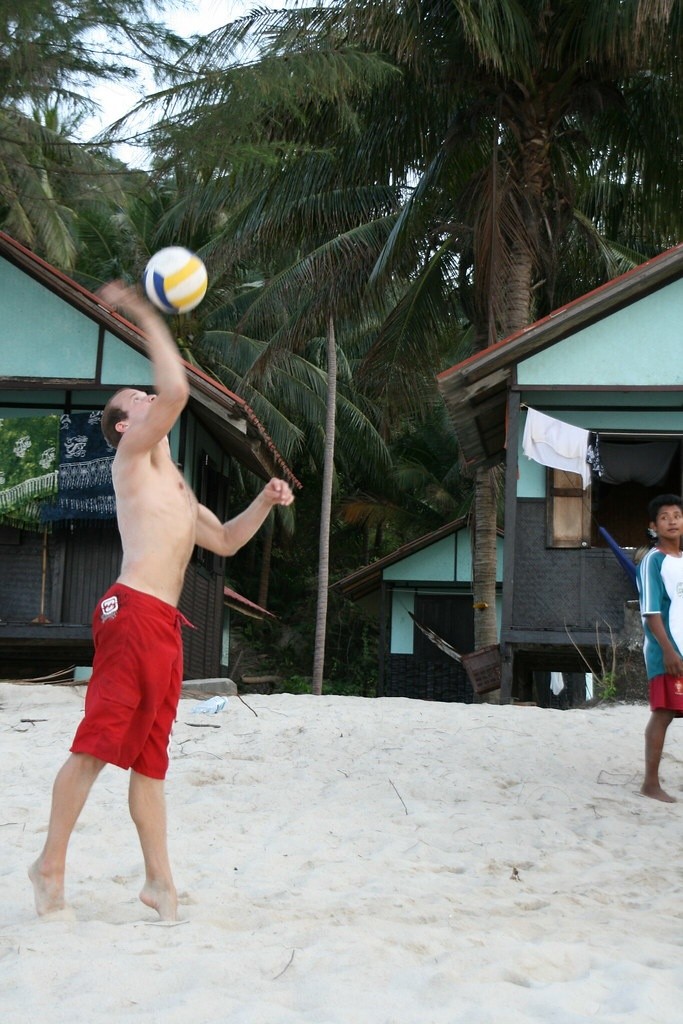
140;245;210;316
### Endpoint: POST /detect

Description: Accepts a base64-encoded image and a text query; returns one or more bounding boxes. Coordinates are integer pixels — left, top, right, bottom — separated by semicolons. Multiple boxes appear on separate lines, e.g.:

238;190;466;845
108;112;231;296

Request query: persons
28;283;295;922
635;493;683;803
